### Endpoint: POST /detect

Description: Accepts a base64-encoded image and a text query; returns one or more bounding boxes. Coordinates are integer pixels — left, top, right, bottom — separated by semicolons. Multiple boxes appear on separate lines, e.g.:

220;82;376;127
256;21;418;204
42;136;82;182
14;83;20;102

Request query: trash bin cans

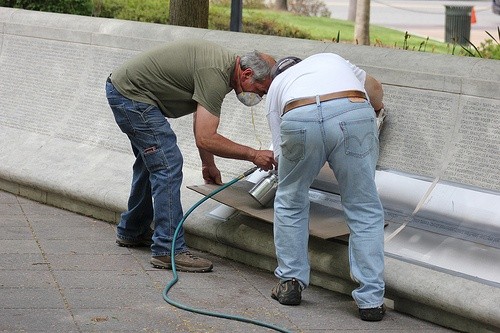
445;4;474;47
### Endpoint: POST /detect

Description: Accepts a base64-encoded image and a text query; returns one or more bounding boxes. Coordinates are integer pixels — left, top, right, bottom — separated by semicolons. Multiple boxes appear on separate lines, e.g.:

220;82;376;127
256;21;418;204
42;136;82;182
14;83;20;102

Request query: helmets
270;56;302;83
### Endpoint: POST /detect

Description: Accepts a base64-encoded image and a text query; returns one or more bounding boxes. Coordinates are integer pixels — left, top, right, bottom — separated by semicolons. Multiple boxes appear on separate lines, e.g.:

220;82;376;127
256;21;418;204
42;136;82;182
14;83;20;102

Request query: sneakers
115;227;158;247
150;251;214;274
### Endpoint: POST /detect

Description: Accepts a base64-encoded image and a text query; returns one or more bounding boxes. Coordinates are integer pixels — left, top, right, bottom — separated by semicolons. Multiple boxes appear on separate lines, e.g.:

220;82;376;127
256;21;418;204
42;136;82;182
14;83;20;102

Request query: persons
104;39;277;273
265;53;387;322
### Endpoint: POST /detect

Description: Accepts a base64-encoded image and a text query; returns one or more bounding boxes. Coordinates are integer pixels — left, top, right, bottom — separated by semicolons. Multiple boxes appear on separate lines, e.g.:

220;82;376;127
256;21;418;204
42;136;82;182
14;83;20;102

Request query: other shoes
270;277;303;306
358;303;387;322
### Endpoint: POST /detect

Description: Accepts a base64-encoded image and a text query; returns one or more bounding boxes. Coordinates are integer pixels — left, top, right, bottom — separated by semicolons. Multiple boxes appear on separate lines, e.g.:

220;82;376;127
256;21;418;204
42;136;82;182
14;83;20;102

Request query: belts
107;73;112;83
283;90;368;115
